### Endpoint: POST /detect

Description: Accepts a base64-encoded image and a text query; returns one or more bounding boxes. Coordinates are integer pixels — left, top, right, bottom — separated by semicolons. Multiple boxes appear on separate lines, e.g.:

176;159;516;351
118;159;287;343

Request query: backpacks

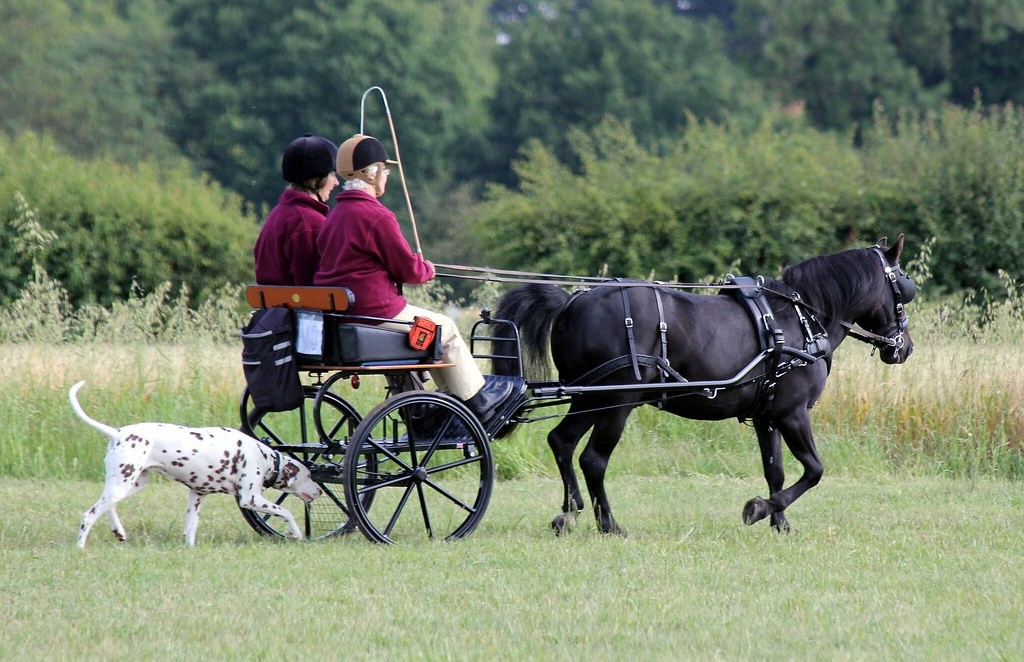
241;308;303;413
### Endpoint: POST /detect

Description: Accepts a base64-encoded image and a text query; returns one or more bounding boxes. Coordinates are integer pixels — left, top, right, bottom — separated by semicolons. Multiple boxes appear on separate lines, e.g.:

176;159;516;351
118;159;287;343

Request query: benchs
245;283;441;367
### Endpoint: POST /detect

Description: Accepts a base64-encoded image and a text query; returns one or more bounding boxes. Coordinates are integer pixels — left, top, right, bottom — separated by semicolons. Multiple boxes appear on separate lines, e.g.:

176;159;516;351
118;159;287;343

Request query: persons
254;133;445;429
314;133;513;434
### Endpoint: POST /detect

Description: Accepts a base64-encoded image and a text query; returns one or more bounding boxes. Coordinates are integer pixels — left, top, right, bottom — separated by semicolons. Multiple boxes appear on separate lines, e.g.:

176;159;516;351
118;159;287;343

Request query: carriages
228;86;919;534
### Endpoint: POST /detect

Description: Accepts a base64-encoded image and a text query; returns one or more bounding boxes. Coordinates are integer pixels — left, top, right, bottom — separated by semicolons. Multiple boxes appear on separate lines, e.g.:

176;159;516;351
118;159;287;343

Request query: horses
483;232;916;539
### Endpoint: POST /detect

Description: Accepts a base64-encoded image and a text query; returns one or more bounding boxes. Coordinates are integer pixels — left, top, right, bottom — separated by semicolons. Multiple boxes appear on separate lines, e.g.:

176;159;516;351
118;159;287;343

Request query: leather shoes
468;379;514;425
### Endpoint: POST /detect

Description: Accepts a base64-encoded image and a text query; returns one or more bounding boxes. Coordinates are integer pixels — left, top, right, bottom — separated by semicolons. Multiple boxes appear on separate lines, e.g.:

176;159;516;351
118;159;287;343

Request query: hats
282;134;338;183
336;132;399;175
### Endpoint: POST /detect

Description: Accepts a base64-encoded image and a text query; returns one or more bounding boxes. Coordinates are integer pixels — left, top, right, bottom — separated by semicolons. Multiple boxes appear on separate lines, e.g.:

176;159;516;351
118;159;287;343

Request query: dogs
67;380;324;552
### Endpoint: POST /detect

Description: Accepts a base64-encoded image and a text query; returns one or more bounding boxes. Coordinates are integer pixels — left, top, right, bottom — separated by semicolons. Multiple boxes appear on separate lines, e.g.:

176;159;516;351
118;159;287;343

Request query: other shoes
415;391;457;438
